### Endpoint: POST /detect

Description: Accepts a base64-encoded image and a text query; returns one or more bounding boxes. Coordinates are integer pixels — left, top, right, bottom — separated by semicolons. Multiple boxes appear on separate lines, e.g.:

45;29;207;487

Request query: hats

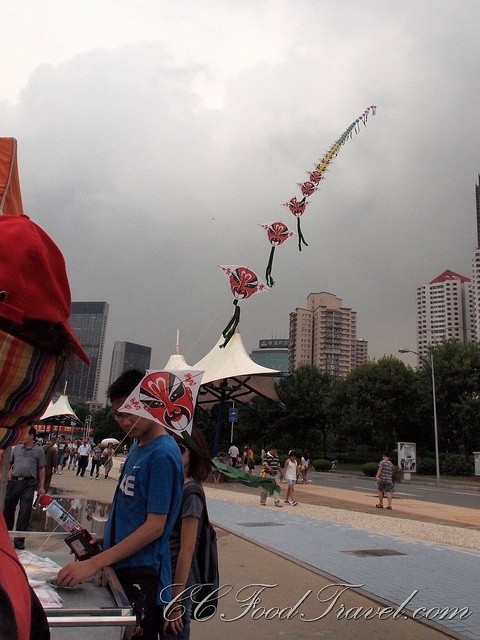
0;214;91;366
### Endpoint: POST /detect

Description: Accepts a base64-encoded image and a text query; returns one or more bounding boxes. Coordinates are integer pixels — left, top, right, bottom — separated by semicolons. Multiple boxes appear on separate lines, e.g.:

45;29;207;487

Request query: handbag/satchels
100;457;107;466
113;565;158;640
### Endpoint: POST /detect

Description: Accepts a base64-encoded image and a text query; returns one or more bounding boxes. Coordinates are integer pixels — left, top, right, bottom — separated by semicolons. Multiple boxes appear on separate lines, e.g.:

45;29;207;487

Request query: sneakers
376;504;383;508
292;501;298;506
275;503;282;507
386;506;392;510
284;501;290;505
260;501;266;506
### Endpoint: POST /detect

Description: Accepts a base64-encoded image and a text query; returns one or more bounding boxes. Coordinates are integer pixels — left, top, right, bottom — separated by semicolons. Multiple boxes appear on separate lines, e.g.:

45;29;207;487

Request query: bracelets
170;597;182;612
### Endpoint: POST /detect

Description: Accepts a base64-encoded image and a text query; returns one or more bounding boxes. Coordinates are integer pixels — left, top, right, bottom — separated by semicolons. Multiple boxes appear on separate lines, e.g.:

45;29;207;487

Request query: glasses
178;444;188;455
113;413;135;421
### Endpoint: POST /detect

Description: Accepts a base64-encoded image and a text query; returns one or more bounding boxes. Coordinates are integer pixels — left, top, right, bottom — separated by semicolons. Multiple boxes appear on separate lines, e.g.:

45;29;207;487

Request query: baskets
64;529;101;561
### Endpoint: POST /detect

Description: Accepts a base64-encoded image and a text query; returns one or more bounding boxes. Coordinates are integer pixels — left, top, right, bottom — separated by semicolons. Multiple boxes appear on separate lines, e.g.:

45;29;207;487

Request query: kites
257;219;300;288
296;181;322;203
306;171;324;187
313;105;377;172
117;369;281;497
218;263;271;349
281;197;311;253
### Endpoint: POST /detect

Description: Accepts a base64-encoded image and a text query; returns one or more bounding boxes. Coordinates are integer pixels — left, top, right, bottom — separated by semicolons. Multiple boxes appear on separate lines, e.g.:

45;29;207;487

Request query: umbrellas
101;437;120;451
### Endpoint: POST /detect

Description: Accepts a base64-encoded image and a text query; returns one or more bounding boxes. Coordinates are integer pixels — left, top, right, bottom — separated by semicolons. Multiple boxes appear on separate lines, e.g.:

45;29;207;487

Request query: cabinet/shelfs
9;530;137;640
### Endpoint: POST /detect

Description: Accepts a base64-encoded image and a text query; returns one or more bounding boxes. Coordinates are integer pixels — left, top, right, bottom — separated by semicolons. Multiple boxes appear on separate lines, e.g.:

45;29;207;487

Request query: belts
12;476;35;481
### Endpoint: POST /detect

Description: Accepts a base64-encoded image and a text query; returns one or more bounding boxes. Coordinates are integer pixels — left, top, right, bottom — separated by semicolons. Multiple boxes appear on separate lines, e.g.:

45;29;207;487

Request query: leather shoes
16;543;25;550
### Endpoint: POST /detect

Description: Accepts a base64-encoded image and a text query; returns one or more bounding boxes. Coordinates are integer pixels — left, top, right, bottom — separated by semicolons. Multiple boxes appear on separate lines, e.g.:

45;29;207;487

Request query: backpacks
177;485;219;620
391;465;404;485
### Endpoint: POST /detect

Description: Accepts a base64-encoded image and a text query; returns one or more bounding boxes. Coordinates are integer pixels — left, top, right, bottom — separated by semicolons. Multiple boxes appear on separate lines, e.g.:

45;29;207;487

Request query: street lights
398;347;442;483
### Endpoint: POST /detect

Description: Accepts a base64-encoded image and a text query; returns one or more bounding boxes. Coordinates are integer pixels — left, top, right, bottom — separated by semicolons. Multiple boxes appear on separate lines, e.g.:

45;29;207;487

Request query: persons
0;215;91;640
76;438;92;476
247;443;255;475
260;444;283;507
242;445;248;471
219;453;231;483
53;435;68;475
90;441;102;480
4;427;47;550
159;428;212;640
55;368;185;640
211;456;222;483
228;442;239;468
32;436;57;510
102;442;115;479
283;451;298;506
66;441;78;470
300;452;310;483
376;452;395;509
